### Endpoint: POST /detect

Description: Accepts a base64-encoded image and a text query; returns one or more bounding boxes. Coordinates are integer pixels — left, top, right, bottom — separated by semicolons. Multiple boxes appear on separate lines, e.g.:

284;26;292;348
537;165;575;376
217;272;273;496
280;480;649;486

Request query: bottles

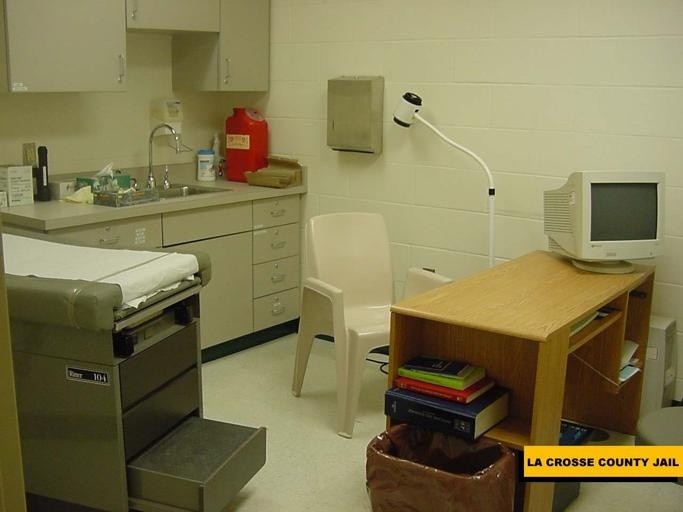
195;148;216;182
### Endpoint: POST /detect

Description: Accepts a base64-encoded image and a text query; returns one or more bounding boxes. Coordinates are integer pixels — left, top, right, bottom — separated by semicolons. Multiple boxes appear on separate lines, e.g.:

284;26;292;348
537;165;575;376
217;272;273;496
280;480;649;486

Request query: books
567;309;600;338
618;339;644;384
382;351;512;443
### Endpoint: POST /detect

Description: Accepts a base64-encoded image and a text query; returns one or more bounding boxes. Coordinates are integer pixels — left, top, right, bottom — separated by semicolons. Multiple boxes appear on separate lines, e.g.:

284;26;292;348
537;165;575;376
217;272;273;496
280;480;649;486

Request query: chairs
289;211;452;439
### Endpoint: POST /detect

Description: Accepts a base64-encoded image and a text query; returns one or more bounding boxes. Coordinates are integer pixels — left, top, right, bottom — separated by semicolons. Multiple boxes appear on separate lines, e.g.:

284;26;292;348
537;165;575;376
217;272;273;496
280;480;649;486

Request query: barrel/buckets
225;107;268;182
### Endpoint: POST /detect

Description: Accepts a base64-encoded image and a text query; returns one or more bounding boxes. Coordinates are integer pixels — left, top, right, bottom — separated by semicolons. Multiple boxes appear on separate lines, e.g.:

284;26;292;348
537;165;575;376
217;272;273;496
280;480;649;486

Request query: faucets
148;123;179;189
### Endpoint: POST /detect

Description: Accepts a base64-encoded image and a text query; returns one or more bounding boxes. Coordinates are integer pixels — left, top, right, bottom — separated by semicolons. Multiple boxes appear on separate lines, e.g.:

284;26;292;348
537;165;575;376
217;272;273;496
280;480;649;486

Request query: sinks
155;185;234;199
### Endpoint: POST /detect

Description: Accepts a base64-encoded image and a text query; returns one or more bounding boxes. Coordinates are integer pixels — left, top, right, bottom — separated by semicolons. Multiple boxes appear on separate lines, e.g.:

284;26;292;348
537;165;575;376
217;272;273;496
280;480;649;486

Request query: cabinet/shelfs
4;0;126;93
172;1;271;92
126;0;219;34
252;194;308;335
160;200;254;358
0;210;161;256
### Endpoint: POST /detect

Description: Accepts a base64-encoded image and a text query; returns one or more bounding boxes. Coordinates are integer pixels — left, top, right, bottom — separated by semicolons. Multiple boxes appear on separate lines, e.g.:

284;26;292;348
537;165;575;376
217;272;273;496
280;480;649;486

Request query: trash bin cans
552;421;592;512
366;423;517;512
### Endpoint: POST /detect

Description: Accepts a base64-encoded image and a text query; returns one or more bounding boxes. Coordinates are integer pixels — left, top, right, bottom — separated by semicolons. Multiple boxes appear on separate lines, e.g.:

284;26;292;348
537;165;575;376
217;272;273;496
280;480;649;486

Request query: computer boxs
638;315;677;419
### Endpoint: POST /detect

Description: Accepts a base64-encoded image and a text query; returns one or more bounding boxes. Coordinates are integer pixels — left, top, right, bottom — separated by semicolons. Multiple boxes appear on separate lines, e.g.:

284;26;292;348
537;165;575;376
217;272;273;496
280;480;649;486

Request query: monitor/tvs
543;171;666;275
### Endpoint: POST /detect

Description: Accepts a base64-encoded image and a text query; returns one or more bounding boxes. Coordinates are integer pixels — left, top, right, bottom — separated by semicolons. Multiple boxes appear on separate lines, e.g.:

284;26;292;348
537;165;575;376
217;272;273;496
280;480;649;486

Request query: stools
633;405;682;485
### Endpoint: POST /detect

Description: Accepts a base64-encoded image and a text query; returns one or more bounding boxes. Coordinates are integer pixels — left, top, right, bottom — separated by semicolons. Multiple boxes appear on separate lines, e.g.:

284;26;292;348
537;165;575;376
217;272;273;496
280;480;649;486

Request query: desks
388;249;655;511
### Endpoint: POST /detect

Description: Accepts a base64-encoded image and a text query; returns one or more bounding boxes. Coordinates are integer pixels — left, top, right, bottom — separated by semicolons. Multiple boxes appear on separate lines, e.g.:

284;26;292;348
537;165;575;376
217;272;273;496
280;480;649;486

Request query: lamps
390;88;498;269
